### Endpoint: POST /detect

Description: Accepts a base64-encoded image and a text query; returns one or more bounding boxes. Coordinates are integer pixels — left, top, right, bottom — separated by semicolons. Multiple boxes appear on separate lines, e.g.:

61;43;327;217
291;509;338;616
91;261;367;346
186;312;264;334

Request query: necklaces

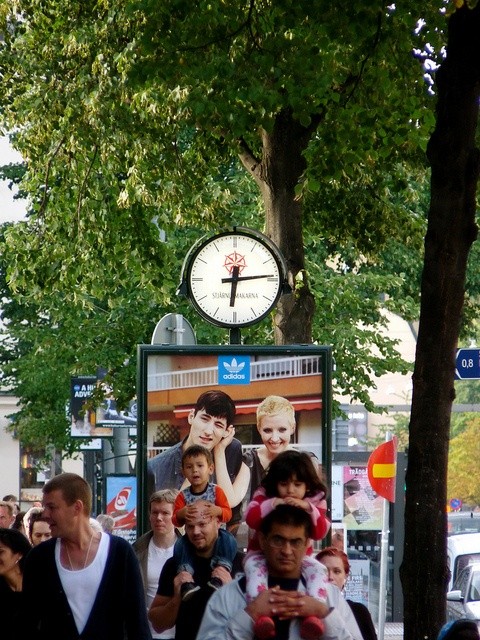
63;531;96;571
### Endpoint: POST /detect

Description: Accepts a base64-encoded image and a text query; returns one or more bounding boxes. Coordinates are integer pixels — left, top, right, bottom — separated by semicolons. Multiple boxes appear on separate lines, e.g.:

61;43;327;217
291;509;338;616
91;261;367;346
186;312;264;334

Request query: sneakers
207;577;221;590
299;616;324;640
181;582;201;601
254;617;275;639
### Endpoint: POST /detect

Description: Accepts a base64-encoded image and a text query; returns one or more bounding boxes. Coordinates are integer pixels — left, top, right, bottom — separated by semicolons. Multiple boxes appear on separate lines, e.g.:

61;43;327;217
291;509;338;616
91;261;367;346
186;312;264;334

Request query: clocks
175;224;293;345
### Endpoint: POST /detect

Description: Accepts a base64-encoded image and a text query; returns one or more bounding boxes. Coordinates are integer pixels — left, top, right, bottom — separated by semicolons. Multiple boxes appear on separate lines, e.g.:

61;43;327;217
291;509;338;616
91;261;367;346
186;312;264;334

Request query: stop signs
367;438;397;503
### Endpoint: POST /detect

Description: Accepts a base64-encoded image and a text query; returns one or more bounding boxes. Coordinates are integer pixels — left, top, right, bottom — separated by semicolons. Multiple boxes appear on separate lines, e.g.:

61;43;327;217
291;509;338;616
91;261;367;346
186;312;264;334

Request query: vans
447;511;480;536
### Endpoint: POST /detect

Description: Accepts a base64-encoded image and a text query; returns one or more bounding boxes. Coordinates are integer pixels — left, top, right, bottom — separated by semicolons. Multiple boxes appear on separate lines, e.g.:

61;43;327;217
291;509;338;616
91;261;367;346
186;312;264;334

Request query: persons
147;387;245;524
2;494;17;507
13;512;25;534
214;394;321;512
22;472;154;640
96;514;115;536
132;488;184;640
244;449;332;640
29;517;52;547
315;546;378;640
0;528;33;639
0;501;16;528
171;444;238;602
194;503;364;640
148;500;246;640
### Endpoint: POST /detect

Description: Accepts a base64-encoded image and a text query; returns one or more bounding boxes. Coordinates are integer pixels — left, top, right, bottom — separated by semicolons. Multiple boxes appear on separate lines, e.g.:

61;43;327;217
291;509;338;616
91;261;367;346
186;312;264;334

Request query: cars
445;532;480;637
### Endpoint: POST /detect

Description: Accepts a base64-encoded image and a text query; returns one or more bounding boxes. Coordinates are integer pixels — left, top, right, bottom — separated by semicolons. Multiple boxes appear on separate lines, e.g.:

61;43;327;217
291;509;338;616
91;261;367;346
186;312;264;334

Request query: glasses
268;537;304;548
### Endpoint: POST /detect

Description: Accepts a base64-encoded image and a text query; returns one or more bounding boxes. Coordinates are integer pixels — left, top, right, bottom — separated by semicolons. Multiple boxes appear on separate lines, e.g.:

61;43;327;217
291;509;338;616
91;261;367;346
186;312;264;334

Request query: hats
1;529;31;555
